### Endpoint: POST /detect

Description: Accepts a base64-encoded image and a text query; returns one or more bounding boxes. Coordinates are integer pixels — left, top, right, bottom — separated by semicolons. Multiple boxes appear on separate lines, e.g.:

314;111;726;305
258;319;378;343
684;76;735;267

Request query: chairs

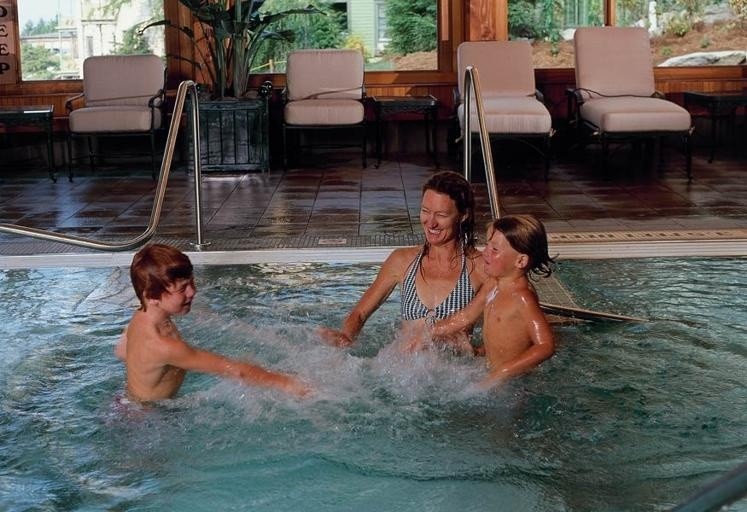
282;48;367;174
60;55;175;181
565;26;690;185
454;40;553;186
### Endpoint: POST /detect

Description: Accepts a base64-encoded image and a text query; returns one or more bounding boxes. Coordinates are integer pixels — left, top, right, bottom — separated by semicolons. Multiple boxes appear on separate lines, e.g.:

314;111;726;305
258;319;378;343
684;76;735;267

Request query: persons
404;213;557;396
100;243;315;424
313;171;499;362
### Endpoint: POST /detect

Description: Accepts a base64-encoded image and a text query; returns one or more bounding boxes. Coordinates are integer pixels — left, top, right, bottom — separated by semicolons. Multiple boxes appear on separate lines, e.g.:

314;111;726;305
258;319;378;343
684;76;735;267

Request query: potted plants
137;0;328;173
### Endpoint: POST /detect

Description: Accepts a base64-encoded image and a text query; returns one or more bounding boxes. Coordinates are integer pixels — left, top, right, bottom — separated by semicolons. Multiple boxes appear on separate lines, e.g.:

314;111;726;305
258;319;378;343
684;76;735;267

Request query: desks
371;94;439;170
0;103;57;182
682;91;746;162
